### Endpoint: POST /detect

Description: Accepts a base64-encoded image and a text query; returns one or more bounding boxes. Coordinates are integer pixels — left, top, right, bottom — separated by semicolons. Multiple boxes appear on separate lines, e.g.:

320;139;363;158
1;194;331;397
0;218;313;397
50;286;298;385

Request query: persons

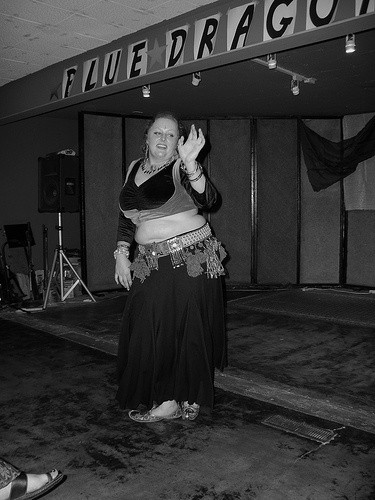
0;458;64;500
113;111;232;429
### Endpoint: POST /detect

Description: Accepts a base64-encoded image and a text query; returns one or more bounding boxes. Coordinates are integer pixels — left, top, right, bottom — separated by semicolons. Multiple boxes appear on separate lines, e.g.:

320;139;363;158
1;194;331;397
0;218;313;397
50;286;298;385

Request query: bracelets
181;162;204;182
114;244;129;260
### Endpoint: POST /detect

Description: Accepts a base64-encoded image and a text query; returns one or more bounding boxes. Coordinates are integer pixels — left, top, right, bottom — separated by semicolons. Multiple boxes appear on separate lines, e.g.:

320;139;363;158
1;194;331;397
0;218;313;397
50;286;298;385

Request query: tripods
41;212;97;309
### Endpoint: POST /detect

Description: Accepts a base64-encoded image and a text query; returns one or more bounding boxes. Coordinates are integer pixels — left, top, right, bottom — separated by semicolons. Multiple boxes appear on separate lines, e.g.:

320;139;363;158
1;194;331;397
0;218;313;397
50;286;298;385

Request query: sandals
6;470;64;500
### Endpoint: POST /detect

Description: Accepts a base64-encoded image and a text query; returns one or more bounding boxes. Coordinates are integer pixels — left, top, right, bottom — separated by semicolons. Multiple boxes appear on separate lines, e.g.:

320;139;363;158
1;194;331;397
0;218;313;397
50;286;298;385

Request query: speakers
38;154;81;213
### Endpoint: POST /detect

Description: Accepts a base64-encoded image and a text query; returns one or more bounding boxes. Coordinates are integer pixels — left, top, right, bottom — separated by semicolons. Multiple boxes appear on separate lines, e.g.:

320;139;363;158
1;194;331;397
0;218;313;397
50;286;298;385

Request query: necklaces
140;157;174;173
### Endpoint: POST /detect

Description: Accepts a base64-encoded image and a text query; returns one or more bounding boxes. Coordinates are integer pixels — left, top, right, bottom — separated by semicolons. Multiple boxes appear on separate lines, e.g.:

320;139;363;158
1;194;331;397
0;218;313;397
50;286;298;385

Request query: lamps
266;53;277;69
192;72;201;86
345;33;356;53
142;85;150;97
291;76;300;95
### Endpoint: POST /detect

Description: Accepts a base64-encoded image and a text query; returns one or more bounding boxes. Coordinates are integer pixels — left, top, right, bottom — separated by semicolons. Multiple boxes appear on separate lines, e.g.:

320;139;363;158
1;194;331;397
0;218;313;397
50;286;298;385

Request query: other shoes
128;402;183;423
182;401;200;421
